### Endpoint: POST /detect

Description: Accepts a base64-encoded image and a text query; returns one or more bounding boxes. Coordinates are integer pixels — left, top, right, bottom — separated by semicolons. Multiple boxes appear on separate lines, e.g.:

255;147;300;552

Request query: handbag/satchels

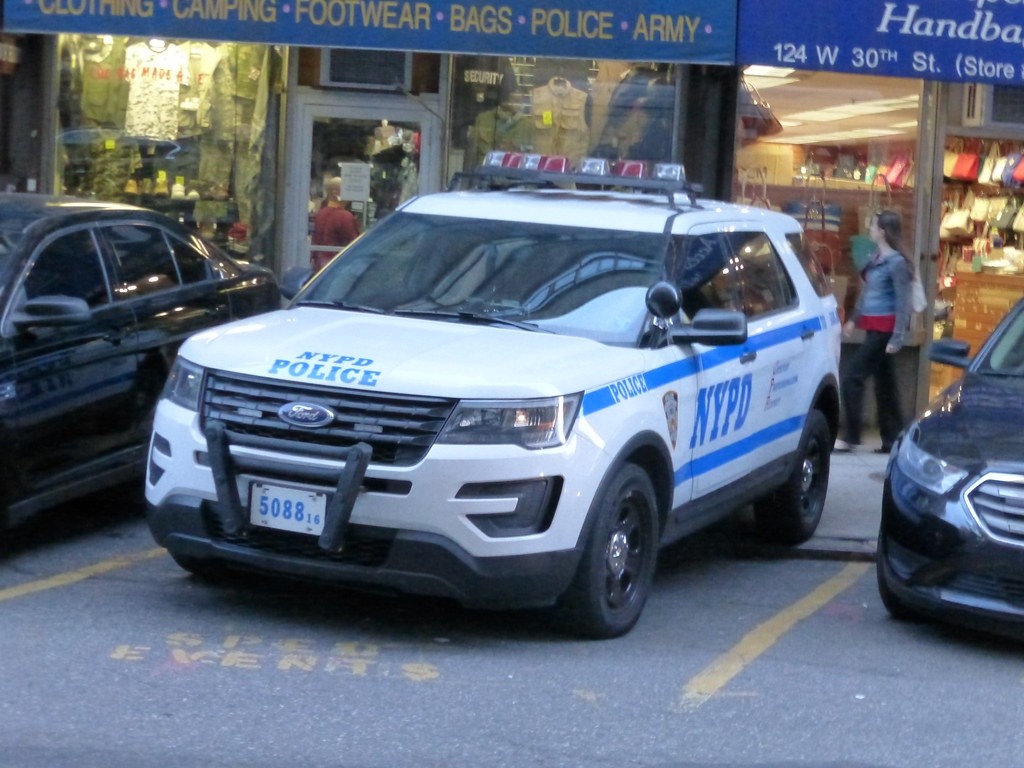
738;167;782;215
857;174;902;238
803;201;840;270
905;277;927;317
785;173;841;233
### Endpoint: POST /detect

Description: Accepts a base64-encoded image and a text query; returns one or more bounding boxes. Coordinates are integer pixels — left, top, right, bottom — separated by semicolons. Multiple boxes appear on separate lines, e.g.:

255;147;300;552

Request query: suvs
141;149;841;636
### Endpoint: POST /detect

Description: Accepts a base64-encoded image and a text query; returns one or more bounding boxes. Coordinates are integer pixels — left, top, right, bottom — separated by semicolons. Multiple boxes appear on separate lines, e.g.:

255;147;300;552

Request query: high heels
830;442;858;455
870;427;903;455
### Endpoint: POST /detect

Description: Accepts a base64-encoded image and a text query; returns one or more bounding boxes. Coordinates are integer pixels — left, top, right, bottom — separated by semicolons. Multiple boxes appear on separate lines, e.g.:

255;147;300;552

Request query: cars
0;193;281;542
873;300;1024;629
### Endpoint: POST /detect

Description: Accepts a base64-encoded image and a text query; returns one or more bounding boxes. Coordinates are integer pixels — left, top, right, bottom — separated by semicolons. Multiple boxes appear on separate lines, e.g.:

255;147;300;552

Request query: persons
311;177;360;270
829;210;916;455
